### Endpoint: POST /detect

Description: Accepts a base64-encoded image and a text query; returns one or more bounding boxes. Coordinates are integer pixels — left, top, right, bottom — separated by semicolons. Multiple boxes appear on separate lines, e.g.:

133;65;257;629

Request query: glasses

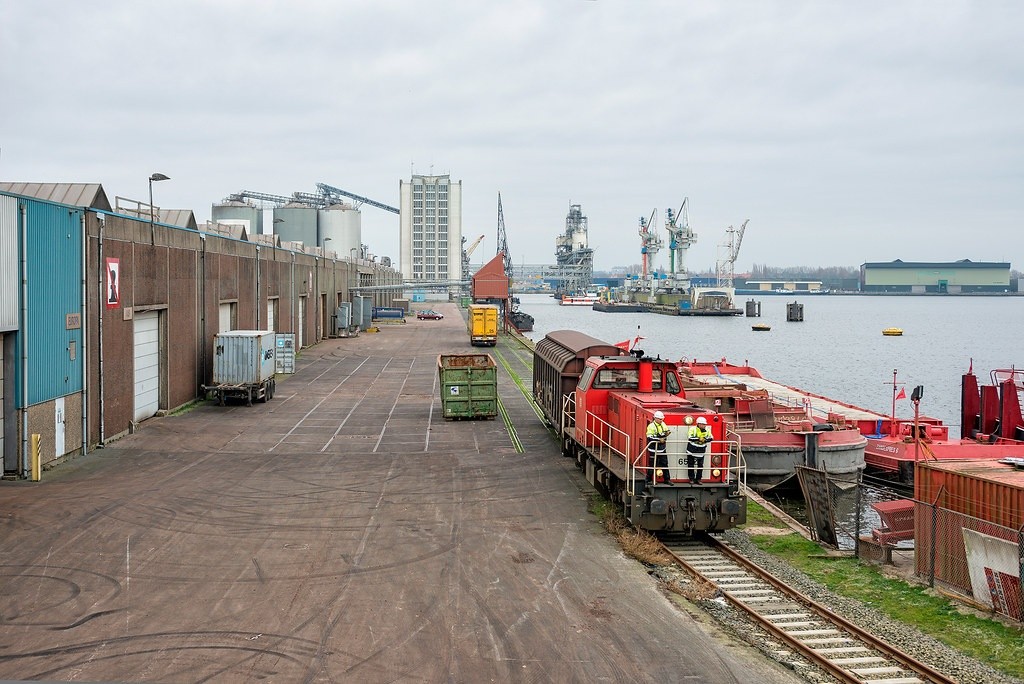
659;419;662;420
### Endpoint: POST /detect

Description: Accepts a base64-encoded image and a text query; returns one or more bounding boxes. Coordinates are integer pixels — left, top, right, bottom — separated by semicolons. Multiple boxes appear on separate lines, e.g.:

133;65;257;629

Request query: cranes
692;218;750;310
656;197;698;292
625;207;664;291
467;234;485;257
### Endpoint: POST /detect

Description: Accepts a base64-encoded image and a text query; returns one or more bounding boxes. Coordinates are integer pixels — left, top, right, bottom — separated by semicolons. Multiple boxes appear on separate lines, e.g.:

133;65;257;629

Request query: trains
532;329;748;543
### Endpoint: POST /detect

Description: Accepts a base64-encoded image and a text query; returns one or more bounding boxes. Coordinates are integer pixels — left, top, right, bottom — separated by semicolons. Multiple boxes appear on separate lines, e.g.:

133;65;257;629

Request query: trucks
467;304;498;347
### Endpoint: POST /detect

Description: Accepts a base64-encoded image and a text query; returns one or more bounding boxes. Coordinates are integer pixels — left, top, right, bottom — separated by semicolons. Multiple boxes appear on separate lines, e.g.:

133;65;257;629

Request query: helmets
653;411;665;419
696;417;707;424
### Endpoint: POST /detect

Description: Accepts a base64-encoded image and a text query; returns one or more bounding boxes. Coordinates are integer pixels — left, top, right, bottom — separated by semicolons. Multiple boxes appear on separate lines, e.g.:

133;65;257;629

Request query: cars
417;309;444;321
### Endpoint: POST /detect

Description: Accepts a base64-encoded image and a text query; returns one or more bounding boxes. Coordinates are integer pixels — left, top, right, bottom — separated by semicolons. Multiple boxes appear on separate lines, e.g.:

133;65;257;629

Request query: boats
752;324;771;331
882;328;902;336
673;382;866;496
677;355;1024;485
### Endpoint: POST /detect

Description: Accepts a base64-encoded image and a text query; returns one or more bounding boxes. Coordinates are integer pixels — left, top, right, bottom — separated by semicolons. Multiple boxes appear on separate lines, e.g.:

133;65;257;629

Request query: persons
686;417;714;484
646;411;676;487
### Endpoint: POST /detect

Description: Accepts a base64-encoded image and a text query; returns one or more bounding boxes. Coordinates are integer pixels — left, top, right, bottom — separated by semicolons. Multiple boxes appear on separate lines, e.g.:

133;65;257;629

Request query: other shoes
664;480;674;485
696;480;702;485
690;480;694;484
647;481;657;485
